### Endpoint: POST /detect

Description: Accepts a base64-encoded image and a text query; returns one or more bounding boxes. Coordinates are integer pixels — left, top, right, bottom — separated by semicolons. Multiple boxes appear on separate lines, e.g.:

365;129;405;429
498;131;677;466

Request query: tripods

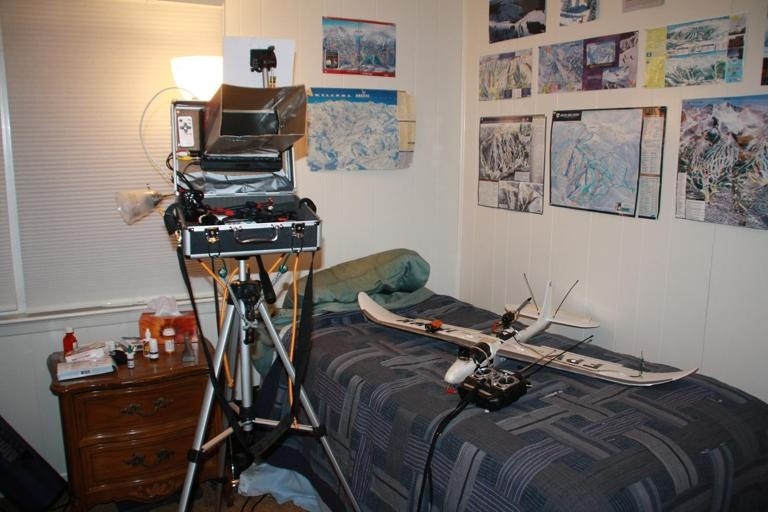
179;259;362;512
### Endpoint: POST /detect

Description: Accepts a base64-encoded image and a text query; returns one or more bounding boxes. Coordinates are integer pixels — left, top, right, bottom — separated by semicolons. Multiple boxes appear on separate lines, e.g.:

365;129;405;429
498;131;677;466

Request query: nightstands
45;332;226;512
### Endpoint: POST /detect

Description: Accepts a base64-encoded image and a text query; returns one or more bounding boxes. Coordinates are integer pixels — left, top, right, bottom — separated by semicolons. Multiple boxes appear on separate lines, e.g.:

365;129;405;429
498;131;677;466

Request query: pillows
284;248;431;306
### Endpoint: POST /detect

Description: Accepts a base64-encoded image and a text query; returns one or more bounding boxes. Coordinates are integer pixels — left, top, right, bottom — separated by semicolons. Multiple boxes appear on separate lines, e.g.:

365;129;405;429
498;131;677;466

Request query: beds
249;248;768;512
